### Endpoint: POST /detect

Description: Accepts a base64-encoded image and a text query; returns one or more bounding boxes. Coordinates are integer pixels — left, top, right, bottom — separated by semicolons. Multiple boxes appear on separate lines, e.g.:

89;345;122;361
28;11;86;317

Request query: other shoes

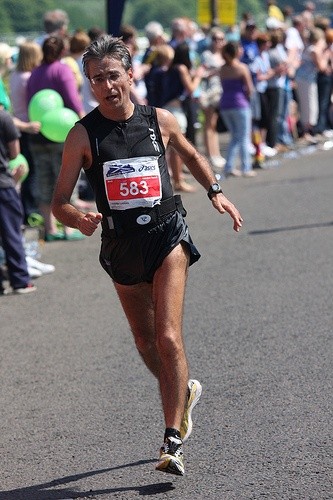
25;255;55;278
174;179;198;192
181;154;268;178
0;282;38;294
46;229;87;241
253;129;333;161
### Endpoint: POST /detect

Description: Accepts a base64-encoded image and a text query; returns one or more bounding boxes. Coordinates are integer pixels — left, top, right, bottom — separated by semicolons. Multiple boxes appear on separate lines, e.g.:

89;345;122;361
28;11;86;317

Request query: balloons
28;89;64;121
40;107;80;143
9;153;29;183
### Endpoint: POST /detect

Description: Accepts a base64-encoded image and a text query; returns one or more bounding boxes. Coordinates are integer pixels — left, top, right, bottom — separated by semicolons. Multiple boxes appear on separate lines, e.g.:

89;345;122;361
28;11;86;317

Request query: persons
50;33;244;476
0;0;333;293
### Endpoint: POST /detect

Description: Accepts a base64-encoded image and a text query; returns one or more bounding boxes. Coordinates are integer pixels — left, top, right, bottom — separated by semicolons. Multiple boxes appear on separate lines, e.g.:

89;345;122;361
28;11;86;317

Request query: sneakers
154;437;184;477
179;379;202;441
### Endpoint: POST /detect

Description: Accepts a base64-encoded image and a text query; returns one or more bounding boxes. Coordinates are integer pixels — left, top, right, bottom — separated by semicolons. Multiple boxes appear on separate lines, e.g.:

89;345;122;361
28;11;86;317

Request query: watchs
207;182;223;199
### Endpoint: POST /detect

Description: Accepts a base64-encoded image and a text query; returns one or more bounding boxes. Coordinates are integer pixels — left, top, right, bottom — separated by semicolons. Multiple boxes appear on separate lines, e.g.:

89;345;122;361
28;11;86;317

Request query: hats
0;43;19;58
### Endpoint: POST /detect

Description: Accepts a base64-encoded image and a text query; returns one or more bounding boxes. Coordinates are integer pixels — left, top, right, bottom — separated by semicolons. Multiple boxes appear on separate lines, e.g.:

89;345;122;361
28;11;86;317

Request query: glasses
89;71;127;88
10;55;17;62
